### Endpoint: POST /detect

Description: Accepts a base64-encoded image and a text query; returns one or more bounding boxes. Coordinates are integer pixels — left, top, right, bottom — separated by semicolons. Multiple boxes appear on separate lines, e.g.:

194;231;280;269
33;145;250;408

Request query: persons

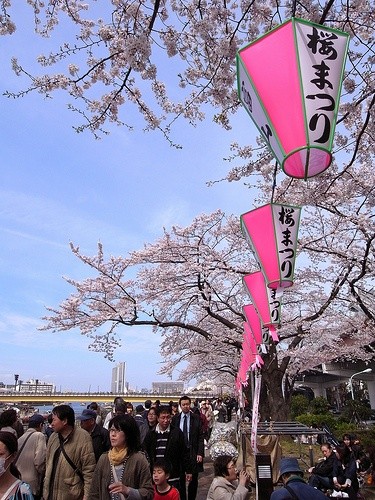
0;395;239;500
309;434;375;500
152;458;180;500
270;457;330;500
207;455;253;500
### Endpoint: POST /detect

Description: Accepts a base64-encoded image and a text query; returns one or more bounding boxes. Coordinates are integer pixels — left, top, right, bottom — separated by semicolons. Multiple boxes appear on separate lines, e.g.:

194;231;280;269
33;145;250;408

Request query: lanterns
240;202;301;291
242;271;283;342
235;16;350;182
236;304;267;396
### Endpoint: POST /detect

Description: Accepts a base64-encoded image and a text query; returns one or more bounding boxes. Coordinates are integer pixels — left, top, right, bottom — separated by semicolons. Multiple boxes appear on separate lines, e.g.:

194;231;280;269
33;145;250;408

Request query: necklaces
111;457;127;500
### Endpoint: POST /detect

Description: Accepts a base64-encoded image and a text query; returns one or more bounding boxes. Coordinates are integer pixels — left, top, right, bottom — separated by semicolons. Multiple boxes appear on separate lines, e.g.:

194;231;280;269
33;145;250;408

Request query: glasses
159;416;172;420
227;465;235;471
148;413;156;416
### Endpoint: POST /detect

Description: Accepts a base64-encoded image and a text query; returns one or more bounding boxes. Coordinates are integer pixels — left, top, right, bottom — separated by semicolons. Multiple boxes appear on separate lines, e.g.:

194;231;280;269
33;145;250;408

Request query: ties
183;415;188;447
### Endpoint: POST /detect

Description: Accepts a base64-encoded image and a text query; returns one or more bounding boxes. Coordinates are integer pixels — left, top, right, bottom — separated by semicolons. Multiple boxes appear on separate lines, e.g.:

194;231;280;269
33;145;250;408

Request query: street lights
350;368;372;400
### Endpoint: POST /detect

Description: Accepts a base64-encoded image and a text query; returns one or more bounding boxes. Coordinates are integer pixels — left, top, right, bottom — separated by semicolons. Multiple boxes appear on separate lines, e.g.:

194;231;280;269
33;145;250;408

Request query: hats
201;401;206;405
78;409;97;420
30;415;45;424
277;458;304;484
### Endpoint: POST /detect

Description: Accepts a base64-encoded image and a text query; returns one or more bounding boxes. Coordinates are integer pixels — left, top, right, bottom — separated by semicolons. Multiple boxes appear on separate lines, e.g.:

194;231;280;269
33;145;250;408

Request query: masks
0;454;13;476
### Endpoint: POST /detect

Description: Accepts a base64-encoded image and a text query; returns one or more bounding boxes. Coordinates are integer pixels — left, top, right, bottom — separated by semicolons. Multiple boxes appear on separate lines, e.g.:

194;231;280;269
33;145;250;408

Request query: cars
328;406;338;414
